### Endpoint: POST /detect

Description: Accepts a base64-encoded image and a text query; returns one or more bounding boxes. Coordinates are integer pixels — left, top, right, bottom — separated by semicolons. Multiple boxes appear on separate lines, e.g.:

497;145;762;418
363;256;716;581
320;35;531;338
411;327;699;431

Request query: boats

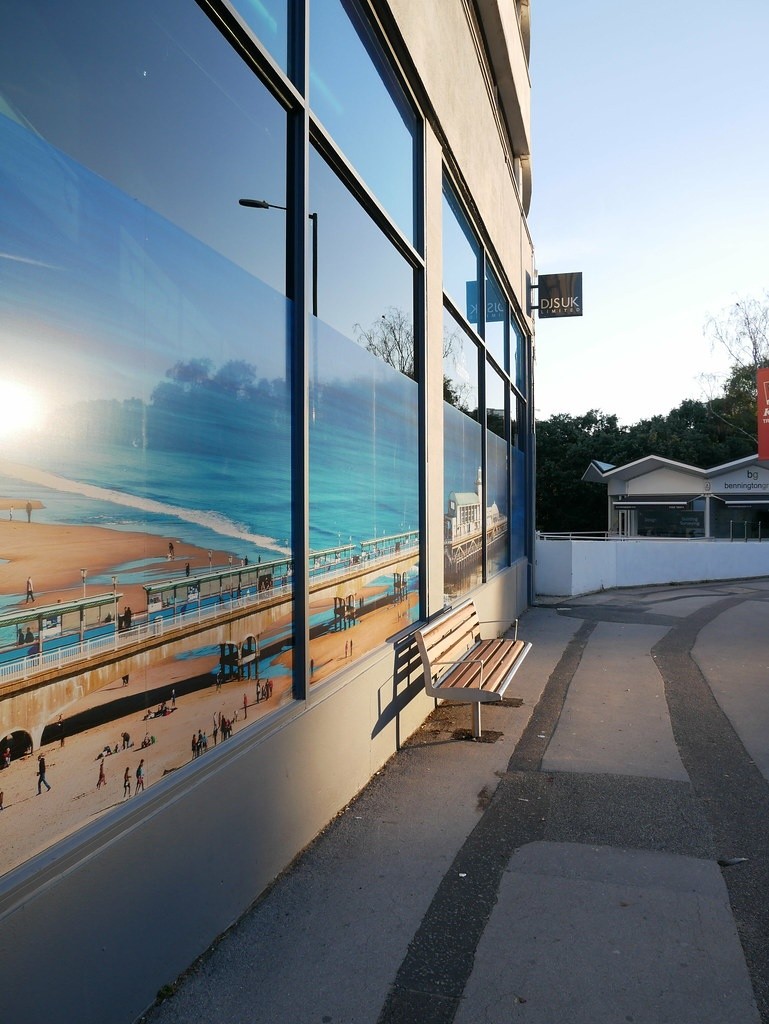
444;466;509;579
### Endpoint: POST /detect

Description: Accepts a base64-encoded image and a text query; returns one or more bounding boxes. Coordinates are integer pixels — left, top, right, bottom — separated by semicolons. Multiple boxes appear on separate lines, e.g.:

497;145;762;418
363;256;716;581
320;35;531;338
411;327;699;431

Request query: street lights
206;548;213;571
337;532;342;547
111;575;120;650
81;568;88;598
229;556;233;598
237;197;317;317
284;538;288;555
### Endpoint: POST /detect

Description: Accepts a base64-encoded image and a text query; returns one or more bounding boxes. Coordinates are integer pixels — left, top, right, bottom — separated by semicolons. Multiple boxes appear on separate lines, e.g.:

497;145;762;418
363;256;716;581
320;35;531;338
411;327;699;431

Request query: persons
9;506;15;521
167;543;174;559
184;563;190;577
345;640;353;658
18;627;34;645
26;577;36;605
96;668;273;797
27;643;39;654
26;501;33;523
0;723;66;810
105;607;131;631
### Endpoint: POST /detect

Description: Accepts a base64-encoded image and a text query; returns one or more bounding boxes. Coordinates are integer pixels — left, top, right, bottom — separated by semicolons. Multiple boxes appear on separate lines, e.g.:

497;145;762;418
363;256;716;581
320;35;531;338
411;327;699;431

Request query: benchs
415;598;533;737
